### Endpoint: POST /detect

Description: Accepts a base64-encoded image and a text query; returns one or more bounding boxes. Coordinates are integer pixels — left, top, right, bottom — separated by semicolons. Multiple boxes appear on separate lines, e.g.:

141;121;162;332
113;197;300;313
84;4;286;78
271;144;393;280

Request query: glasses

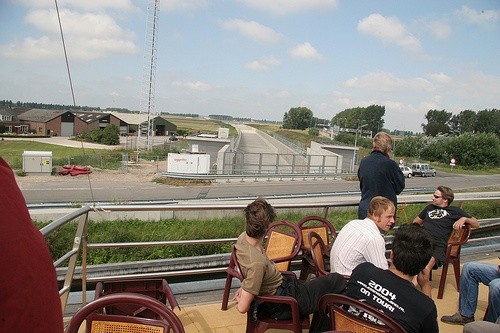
433;195;445;198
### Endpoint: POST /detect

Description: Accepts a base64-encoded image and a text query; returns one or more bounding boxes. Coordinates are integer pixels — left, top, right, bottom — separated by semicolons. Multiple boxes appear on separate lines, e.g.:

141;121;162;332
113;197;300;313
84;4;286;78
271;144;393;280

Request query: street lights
353;124;369;165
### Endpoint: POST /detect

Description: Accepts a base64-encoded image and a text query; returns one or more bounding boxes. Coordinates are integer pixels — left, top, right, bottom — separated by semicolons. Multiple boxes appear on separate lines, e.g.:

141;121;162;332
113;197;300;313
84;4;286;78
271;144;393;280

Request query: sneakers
441;313;474;325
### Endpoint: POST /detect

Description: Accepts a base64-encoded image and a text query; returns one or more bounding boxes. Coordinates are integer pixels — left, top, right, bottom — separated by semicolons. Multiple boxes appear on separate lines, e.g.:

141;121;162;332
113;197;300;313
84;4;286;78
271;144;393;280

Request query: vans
412;163;437;177
398;166;413;178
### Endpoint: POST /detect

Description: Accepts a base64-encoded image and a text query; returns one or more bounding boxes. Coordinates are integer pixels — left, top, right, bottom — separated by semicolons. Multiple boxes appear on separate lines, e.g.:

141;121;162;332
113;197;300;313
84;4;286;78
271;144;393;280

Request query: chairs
64;292;186;333
232;246;312;333
294;215;336;279
318;294;409;333
430;224;471;299
221;219;302;311
94;279;181;320
308;231;330;277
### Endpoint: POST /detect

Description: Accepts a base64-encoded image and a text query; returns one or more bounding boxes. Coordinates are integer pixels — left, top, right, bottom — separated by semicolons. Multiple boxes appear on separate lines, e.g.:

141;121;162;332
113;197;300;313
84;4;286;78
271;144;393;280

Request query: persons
441;255;500;333
411;186;481;288
231;199;345;333
345;223;439;333
330;196;395;283
357;135;405;231
